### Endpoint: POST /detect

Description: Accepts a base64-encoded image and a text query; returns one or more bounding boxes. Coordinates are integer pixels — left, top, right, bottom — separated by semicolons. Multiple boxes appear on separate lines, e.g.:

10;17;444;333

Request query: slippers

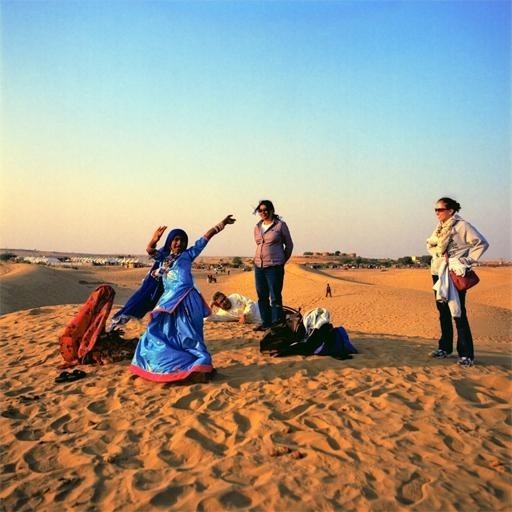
54;369;87;383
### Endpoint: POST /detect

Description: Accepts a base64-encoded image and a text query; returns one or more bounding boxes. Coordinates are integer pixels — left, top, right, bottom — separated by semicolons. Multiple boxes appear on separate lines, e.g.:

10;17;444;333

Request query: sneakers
429;349;453;359
456;356;476;369
253;321;282;331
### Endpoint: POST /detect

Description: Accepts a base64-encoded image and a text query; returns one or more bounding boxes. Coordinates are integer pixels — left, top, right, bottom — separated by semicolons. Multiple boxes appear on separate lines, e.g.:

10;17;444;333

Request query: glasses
434;207;452;213
258;208;270;213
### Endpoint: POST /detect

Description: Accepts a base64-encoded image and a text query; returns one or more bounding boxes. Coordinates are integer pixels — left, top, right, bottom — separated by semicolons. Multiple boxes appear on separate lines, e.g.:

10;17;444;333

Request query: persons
107;213;238;384
423;196;490;370
325;283;333;297
200;291;290;325
58;284;140;366
251;199;294;333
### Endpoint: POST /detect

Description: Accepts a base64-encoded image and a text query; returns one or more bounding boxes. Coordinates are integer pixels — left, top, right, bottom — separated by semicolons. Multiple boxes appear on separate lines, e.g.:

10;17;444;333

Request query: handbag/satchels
449;269;480;292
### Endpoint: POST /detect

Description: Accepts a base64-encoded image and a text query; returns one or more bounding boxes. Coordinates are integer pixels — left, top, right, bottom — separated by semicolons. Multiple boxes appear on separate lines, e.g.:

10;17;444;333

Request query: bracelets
213;223;225;233
